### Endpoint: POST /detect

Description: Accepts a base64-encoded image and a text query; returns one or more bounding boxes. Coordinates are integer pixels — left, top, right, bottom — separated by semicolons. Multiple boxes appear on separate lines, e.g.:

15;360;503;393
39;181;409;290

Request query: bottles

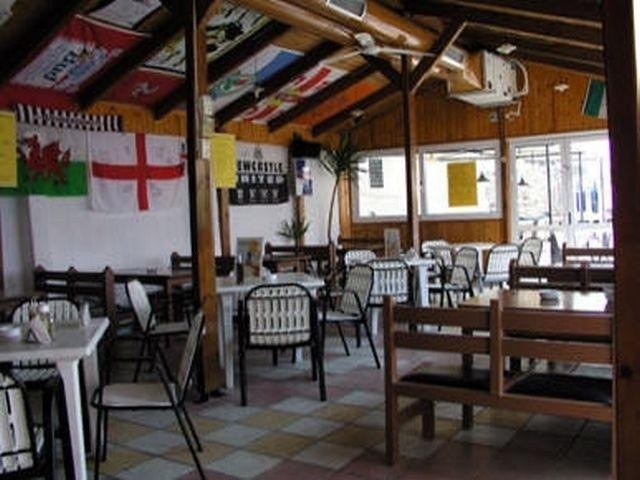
79;300;91;326
233;249;255;289
34;302;55;339
236;160;285;186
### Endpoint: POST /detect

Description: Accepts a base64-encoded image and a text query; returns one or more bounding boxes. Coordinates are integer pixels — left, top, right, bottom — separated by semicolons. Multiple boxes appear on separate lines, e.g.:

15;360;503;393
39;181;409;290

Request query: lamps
496;34;518;55
249;54;266;99
552;71;570;93
350;83;365;117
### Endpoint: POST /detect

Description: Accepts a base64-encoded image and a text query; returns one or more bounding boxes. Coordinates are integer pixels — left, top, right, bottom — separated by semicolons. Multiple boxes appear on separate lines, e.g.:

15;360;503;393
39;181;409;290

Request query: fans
318;32;436;66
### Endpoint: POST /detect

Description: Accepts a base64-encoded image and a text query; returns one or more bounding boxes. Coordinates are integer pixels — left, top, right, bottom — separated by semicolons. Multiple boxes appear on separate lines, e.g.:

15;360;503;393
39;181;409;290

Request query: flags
578;77;608;120
0;109;16;189
0;120;89;198
446;161;478;210
0;2;350;129
88;131;188;215
210;130;240;189
229;142;290;207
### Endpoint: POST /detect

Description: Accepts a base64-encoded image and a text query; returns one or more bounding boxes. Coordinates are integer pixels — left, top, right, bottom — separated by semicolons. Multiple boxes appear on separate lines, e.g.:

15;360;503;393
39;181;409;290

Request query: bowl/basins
1;324;22;339
539;289;559;299
540;298;560;307
262;275;279;283
